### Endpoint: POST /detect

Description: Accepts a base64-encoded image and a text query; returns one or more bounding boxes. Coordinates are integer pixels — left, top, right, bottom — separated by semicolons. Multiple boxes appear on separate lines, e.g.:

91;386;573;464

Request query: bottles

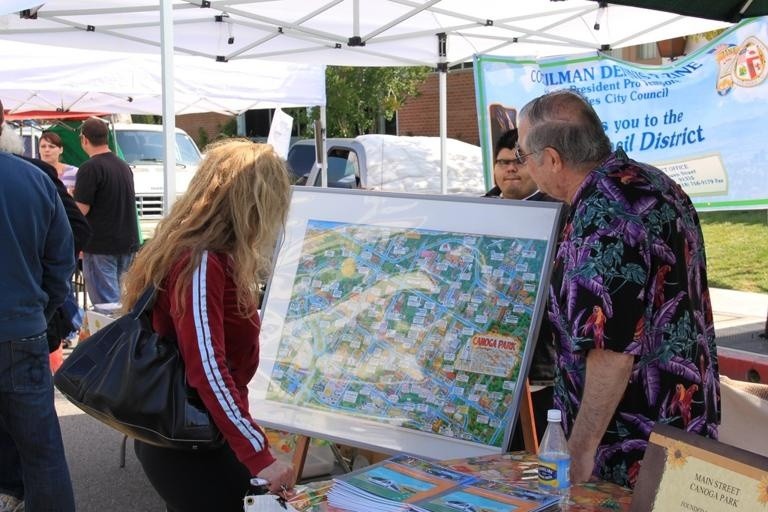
537;408;572;512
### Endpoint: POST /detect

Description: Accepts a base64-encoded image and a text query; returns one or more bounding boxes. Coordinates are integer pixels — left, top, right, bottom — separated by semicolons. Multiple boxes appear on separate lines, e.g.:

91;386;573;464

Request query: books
284;449;635;512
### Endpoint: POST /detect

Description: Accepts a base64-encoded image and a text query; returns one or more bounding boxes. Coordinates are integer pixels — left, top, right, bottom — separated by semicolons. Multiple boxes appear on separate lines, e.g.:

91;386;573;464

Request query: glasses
514;147;544;164
496;159;525;166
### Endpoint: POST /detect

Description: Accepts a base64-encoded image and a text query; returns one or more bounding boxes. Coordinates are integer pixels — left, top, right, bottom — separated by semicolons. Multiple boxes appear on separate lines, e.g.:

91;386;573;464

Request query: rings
280;484;288;490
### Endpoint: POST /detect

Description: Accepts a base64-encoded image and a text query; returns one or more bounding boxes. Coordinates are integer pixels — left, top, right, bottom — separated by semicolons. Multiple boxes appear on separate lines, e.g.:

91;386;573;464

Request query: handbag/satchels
52;311;226;451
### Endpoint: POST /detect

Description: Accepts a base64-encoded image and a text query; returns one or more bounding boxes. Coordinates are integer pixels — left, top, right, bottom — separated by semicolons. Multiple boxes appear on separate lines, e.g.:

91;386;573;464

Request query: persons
113;135;299;512
71;117;142;309
36;131;80;194
0;99;77;510
0;123;94;357
479;130;572;451
512;87;722;491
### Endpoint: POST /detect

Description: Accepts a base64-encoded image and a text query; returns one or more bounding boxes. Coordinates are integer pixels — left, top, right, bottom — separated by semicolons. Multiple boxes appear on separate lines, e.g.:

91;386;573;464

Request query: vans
12;121;207;243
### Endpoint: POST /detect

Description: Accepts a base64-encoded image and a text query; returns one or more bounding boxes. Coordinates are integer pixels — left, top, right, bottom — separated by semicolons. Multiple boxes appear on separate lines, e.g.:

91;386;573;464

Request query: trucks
282;133;370;191
